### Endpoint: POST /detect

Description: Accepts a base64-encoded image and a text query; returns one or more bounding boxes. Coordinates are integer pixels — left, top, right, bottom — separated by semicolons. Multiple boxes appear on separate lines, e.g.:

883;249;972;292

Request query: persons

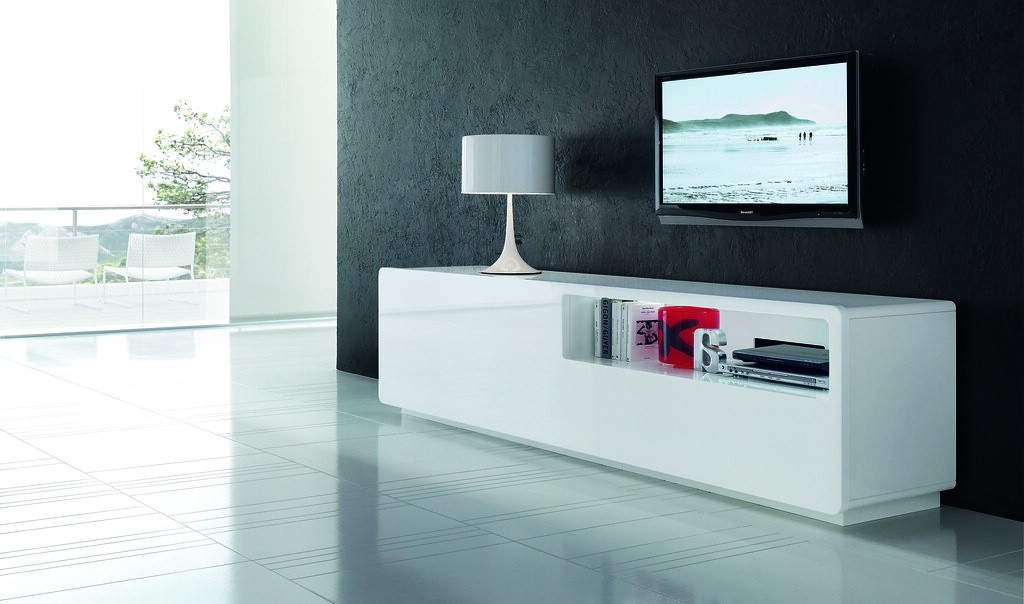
799;132;812;142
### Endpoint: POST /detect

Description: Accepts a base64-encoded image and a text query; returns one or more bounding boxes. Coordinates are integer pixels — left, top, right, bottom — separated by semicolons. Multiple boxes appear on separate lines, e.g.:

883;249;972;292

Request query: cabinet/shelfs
377;265;955;524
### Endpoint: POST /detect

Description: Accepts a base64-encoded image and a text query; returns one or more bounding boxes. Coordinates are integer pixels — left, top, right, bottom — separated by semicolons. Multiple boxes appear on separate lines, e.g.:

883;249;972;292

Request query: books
594;298;659;364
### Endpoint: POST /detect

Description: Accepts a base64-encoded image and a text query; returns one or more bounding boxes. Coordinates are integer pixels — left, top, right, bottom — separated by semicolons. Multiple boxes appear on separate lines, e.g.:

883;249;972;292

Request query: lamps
462;134;556;274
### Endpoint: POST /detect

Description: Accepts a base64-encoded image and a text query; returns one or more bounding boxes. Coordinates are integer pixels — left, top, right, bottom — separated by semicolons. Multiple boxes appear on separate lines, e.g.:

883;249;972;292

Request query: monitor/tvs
653;50;864;228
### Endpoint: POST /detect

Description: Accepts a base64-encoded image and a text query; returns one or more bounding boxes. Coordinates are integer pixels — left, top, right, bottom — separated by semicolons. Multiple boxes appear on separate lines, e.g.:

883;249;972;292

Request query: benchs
3;234;99;283
101;232;196;283
